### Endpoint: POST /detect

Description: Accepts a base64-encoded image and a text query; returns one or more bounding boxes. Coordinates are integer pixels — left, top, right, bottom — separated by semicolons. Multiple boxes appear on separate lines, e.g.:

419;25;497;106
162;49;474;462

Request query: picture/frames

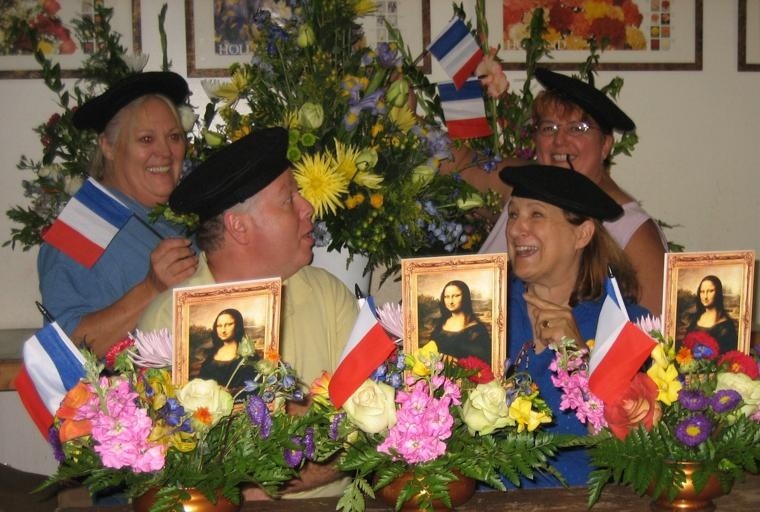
660;248;757;388
735;1;759;74
400;251;510;393
476;1;708;72
169;274;287;419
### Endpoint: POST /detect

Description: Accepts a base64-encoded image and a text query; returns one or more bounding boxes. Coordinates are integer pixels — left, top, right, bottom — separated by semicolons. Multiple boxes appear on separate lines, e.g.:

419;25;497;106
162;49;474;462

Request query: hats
499;164;622;221
169;127;292;223
534;68;635;132
69;72;188;134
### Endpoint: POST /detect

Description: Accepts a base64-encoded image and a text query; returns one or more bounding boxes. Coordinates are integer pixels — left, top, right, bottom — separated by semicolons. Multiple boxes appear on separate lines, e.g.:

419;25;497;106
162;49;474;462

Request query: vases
129;480;245;512
643;458;735;510
365;458;478;511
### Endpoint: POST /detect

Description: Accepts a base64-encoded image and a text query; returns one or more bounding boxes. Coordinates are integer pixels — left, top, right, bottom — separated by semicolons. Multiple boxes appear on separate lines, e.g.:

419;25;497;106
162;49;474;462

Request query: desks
245;472;756;510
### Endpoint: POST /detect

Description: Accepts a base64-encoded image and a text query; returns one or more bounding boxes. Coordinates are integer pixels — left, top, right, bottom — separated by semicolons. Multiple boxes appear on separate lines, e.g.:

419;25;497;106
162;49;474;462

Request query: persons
430;280;490;365
36;68;198;364
474;163;670;493
133;123;364;404
196;309;258;403
685;275;737;358
438;53;666;324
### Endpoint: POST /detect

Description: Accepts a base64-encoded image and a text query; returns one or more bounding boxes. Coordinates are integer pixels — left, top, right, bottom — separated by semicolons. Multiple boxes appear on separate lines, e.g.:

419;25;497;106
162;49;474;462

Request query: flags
587;277;659;406
43;177;130;268
328;300;397;408
12;321;95;441
437;78;493;140
426;15;483;89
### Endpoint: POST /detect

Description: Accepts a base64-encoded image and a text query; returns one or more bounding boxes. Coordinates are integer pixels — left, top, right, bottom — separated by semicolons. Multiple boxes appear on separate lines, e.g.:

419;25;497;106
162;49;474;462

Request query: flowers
1;1;637;285
338;295;597;512
537;313;759;511
30;326;355;512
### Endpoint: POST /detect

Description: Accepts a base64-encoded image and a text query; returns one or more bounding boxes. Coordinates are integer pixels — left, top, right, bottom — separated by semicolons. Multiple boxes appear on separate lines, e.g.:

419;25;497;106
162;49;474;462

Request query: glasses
533;120;602;136
